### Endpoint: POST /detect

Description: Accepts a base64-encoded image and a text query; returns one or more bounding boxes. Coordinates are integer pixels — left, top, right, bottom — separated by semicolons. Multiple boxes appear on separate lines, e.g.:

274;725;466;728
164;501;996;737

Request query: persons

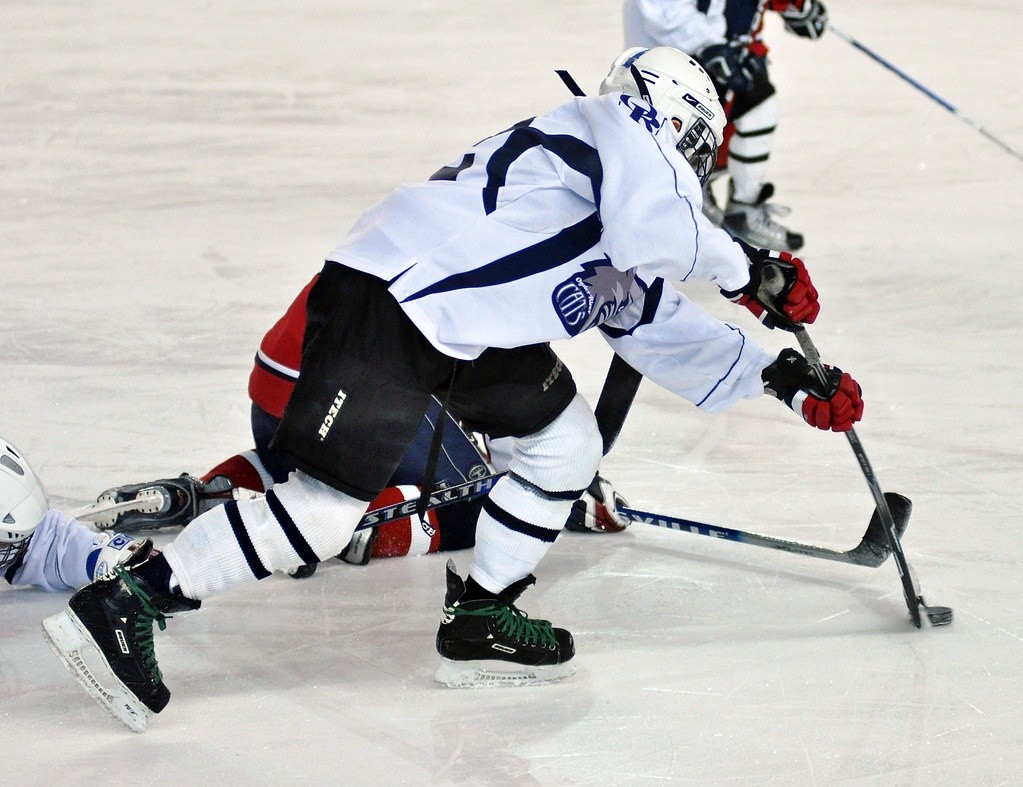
0;275;630;592
622;0;829;251
42;48;864;733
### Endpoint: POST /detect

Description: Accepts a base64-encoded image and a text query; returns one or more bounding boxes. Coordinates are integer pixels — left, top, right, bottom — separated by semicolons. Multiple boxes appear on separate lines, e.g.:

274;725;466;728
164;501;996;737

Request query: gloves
701;41;760;92
87;531;162;582
564;474;633;533
781;0;825;38
720;236;820;332
762;348;864;432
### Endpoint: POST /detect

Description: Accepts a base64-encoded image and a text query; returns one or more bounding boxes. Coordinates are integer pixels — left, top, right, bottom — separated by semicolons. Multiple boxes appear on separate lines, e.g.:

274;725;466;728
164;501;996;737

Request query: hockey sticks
824;24;1023;163
757;258;954;633
603;489;912;567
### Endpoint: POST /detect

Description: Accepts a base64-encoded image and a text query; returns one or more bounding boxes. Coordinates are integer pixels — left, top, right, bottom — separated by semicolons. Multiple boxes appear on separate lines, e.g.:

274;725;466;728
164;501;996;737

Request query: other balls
907;605;953;628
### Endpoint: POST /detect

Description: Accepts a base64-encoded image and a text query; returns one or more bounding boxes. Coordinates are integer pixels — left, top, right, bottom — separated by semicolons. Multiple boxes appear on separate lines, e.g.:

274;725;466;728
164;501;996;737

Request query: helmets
599;46;727;187
0;439;48;579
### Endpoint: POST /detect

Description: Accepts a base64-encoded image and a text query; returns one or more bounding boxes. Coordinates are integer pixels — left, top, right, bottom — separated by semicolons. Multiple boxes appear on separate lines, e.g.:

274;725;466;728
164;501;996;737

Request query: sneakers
40;537;201;735
433;558;577;688
721;179;802;251
71;472;233;537
701;183;724;225
